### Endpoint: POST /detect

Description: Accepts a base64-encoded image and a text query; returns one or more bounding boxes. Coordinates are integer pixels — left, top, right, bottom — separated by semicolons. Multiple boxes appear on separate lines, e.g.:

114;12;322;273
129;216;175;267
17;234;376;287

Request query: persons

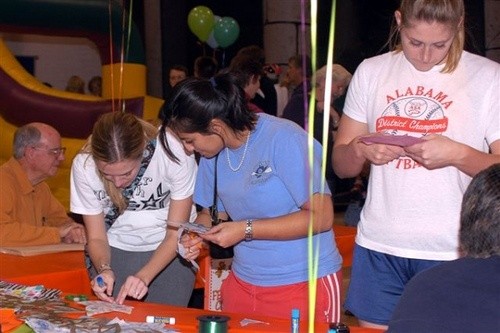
66;75;102;97
70;112;203;307
159;72;344;324
333;0;500;331
384;163;500;333
166;48;369;224
0;122;87;248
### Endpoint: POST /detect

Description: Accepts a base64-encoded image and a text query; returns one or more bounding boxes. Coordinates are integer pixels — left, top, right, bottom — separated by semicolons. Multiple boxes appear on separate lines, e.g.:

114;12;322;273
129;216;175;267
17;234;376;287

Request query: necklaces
226;131;250;172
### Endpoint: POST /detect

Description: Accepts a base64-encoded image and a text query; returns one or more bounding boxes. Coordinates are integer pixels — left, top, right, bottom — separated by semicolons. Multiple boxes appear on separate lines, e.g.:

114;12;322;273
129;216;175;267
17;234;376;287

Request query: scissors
189;233;208;249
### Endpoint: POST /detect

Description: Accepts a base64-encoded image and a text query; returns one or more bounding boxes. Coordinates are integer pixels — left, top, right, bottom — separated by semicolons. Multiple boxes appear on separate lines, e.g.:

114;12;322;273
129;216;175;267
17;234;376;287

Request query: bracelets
97;263;111;274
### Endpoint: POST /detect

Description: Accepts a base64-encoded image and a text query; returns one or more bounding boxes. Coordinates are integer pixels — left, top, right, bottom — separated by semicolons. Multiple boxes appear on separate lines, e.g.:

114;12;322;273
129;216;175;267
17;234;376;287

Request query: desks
0;223;358;298
0;291;384;332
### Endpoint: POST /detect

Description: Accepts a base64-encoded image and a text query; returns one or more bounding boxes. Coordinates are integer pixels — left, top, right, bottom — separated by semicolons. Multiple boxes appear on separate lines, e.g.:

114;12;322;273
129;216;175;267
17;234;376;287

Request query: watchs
245;219;254;242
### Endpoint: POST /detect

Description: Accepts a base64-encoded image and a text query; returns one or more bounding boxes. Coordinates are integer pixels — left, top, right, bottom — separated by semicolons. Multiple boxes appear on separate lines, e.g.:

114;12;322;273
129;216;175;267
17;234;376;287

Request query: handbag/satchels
84;242;93;282
209;152;235;259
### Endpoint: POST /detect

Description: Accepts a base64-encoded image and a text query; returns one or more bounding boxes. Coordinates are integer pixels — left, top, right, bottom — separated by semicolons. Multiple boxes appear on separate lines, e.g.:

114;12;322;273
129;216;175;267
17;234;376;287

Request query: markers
292;309;299;333
97;277;105;288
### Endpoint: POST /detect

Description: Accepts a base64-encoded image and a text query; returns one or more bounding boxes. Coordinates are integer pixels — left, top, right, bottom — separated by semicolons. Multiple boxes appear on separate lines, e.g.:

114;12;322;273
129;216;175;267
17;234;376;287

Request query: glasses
32;148;66;160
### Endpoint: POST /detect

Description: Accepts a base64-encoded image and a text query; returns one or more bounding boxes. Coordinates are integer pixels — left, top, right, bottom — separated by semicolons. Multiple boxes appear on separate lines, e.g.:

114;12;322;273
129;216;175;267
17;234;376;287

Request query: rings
189;247;193;253
135;292;140;296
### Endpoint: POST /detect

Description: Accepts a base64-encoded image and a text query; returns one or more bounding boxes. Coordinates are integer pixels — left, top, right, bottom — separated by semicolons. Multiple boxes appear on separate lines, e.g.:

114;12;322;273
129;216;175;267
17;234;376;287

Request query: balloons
188;6;215;41
207;15;220;49
214;17;240;49
